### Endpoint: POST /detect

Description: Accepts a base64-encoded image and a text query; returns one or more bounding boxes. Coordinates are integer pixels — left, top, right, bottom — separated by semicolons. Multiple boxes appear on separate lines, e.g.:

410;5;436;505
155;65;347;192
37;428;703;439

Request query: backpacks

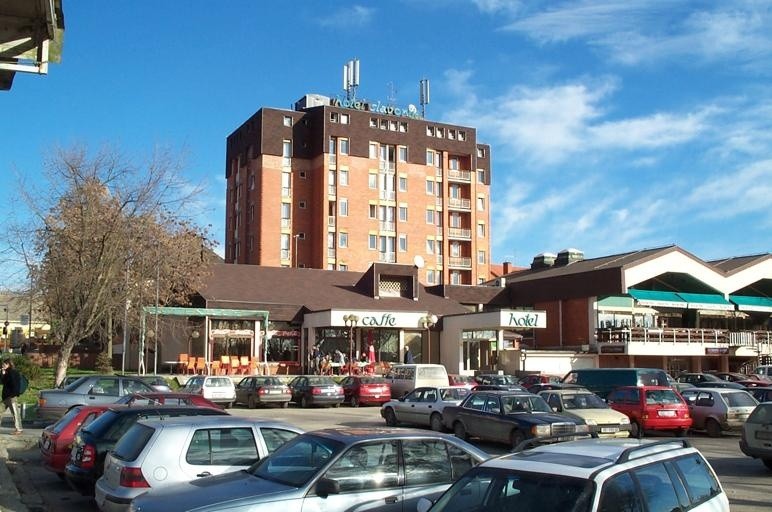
17;370;28;395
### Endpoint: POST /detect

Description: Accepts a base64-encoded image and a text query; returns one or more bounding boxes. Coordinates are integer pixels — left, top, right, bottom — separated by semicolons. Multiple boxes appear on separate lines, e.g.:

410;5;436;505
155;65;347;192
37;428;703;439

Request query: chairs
379;360;390;375
218;429;240;448
341;448;368;471
176;354;257;376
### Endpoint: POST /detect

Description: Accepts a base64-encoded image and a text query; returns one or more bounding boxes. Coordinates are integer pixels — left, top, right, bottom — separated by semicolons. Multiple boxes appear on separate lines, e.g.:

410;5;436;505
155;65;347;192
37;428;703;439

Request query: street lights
340;313;359;376
186;330;200;367
420;313;438;364
144;326;155;374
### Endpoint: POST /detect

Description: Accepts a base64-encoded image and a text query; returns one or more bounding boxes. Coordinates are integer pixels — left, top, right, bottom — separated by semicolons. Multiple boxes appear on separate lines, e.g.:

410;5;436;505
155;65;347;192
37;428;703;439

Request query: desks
331;362;369;375
163;360;279;374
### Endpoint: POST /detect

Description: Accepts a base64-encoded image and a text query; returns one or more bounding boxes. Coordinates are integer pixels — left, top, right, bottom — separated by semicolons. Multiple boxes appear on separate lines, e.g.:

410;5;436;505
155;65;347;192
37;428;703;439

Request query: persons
361;352;368;362
0;358;23;434
404;345;412;364
620;321;629;341
310;345;349;374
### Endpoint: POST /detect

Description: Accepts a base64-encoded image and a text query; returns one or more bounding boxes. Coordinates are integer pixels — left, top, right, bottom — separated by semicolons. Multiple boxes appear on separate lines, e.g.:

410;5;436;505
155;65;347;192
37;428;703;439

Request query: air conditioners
494;277;506;287
